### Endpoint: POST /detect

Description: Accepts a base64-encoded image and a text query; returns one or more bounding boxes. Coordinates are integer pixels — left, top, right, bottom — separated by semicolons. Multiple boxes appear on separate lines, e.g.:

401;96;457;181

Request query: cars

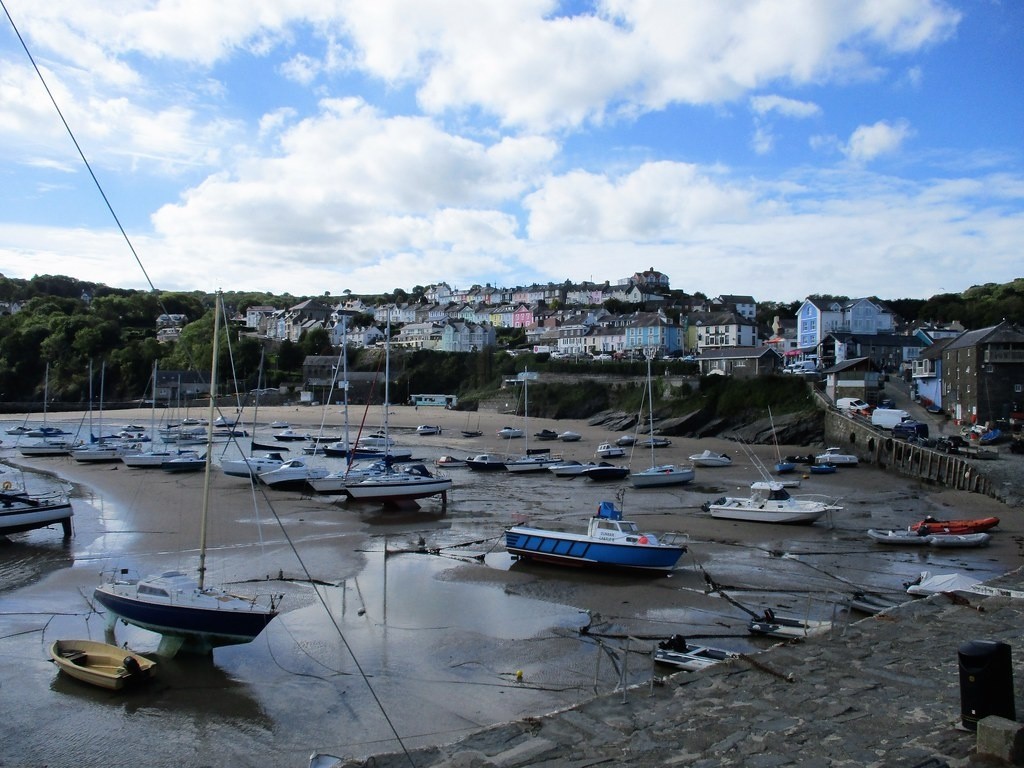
876;399;895;409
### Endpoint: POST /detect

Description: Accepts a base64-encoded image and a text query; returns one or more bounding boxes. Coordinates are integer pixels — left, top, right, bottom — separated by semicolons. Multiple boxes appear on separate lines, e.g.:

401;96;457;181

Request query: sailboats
93;286;285;649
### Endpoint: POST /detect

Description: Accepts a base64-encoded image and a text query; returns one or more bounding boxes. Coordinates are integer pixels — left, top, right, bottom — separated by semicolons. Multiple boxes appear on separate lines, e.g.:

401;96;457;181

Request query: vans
891;423;928;440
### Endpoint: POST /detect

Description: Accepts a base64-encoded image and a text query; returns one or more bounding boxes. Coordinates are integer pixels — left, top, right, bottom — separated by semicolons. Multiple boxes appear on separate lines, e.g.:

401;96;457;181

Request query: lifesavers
3;481;11;488
597;504;601;515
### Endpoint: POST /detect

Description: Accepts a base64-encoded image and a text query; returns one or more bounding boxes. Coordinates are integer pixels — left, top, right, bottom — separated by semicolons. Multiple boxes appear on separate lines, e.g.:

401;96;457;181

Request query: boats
0;481;75;539
47;638;160;689
839;589;901;614
747;607;845;644
4;307;859;515
866;524;993;549
913;515;1001;535
504;487;690;572
709;433;844;523
654;633;750;672
979;429;1002;445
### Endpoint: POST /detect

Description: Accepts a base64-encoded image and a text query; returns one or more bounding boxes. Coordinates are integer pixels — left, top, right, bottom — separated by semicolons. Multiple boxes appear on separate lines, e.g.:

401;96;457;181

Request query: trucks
871;408;916;429
836;397;871;414
782;361;815;374
